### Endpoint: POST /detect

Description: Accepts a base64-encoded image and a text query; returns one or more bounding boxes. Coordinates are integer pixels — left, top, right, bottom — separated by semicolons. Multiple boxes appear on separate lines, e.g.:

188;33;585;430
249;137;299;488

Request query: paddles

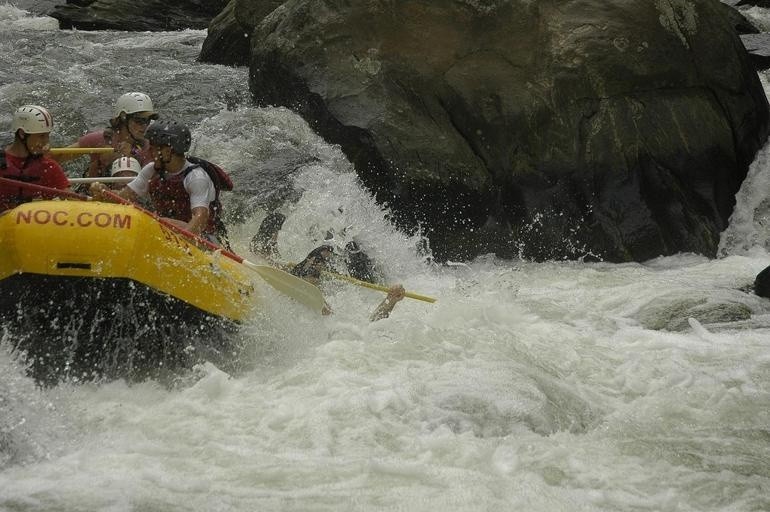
100;186;324;312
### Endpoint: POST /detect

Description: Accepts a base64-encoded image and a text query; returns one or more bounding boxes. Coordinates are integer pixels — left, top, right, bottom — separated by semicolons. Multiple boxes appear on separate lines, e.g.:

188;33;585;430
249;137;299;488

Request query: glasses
126;115;152;125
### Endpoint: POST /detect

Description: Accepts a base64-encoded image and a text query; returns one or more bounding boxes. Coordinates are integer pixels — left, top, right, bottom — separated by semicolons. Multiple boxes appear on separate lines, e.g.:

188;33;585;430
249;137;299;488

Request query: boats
0;199;278;390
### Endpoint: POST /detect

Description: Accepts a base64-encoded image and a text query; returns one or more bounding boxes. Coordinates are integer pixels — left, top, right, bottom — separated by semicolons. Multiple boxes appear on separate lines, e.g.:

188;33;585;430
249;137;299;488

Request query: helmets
11;104;53;134
111;156;141;178
143;119;191;155
115;92;154;119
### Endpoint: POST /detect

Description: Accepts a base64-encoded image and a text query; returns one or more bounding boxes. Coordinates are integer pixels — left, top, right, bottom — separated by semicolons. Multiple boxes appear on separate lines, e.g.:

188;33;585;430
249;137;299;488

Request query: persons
61;90;162;180
1;105;74;211
91;117;237;258
106;151;155;213
251;215;405;325
